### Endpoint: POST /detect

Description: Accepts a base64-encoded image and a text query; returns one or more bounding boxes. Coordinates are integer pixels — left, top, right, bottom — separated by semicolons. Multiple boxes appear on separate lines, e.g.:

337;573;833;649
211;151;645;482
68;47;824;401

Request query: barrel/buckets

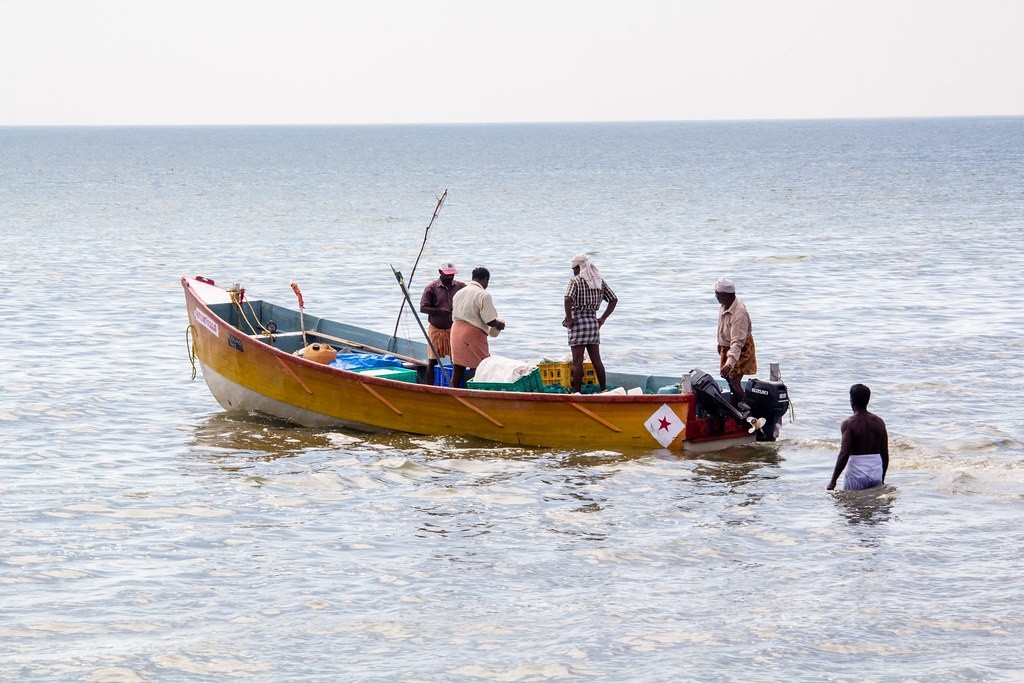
303;342;338;364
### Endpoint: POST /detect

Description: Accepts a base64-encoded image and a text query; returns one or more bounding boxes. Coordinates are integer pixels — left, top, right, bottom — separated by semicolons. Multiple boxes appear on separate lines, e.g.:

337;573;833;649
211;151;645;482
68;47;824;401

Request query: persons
450;266;505;388
715;276;757;403
563;252;619;394
420;262;467;387
827;384;890;491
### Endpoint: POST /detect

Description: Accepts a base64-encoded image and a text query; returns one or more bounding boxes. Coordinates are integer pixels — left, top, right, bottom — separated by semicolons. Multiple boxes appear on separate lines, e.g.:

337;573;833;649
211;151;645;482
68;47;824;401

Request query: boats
180;274;797;458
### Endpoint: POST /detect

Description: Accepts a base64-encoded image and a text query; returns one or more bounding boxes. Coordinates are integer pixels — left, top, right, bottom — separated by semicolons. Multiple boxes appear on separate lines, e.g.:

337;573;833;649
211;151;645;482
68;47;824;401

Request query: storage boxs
538;363;599;389
434;364;470;388
348;366;417;383
467;367;543;392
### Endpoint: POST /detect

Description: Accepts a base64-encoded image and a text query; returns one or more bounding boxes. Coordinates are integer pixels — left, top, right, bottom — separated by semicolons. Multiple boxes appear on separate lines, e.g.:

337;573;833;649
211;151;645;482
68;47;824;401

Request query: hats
439;261;457;275
715;279;736;294
